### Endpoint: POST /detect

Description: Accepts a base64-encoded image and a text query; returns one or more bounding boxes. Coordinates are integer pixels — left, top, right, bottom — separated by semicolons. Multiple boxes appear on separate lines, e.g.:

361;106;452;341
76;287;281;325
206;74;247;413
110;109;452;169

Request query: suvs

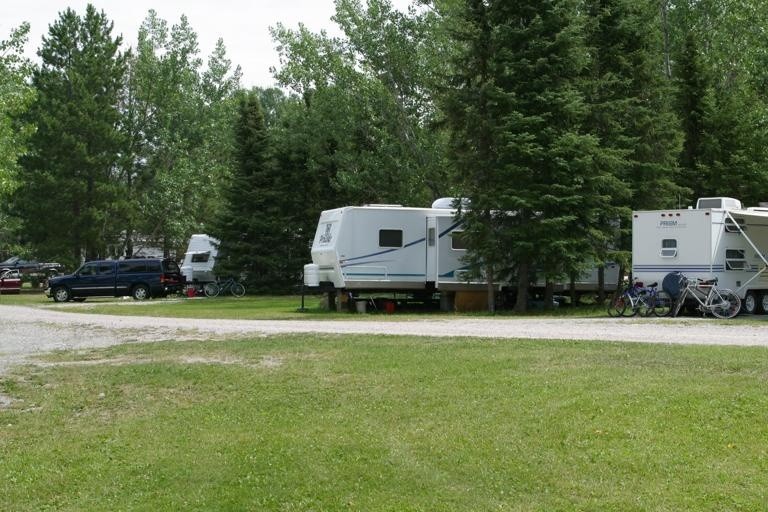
0;268;22;295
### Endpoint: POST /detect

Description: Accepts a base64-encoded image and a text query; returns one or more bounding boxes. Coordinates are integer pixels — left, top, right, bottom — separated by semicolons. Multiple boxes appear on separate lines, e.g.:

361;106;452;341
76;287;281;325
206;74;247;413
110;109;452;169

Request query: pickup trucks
0;256;67;287
45;257;185;300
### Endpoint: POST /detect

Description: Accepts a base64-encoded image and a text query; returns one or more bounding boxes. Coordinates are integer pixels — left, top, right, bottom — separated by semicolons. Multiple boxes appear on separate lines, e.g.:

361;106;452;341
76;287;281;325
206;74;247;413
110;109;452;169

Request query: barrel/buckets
354;299;367;313
304;263;320;287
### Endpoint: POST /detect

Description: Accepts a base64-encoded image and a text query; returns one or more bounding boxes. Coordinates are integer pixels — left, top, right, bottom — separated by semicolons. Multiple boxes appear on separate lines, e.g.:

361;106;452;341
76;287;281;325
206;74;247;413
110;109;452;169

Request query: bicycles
204;271;248;298
670;271;742;319
605;270;676;318
